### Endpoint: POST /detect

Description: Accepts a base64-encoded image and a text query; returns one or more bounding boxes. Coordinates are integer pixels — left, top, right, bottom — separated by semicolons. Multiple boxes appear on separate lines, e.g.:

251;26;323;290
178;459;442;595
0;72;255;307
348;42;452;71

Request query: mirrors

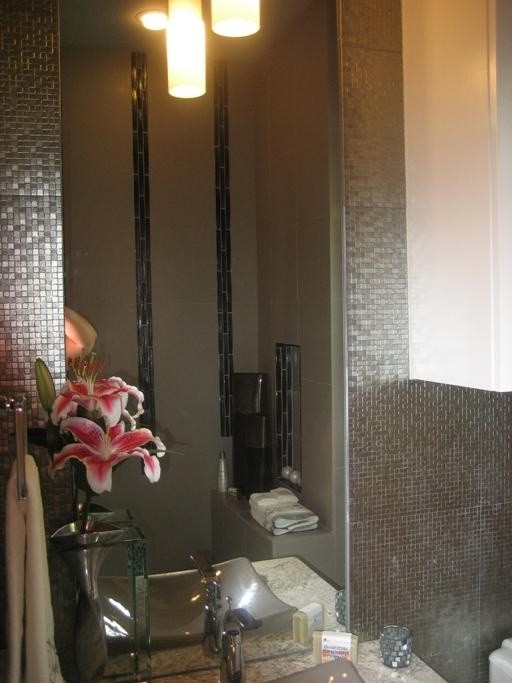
59;0;347;628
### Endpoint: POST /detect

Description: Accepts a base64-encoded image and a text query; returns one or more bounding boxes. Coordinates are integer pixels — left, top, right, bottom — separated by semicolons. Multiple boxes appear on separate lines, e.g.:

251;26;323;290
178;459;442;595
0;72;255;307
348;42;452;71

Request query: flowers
28;346;186;528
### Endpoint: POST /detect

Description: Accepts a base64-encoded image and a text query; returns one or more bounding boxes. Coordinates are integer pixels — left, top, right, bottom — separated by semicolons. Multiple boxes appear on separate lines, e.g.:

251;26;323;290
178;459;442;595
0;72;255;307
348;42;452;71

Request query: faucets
187;550;223;658
219;596;264;682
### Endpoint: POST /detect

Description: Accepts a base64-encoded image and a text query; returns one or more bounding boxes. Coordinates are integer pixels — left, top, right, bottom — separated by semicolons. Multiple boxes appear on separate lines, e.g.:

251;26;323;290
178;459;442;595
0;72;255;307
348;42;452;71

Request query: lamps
210;0;261;37
135;0;206;98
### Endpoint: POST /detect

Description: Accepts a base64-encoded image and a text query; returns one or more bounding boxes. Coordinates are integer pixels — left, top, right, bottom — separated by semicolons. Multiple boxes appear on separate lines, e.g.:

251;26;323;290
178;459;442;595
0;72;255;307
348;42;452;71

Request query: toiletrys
215;448;230;492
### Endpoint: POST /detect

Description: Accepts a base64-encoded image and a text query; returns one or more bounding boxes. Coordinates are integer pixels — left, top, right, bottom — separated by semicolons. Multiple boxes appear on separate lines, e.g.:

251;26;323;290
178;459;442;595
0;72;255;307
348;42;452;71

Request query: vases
46;518;126;683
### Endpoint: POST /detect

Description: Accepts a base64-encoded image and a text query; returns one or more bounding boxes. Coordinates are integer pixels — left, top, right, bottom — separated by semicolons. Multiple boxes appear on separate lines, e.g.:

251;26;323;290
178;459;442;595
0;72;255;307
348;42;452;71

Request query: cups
335;587;346;625
379;625;414;669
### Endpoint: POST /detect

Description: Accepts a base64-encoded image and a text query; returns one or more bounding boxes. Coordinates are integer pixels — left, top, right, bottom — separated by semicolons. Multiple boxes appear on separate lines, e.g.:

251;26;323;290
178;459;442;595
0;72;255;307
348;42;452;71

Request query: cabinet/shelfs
400;0;511;394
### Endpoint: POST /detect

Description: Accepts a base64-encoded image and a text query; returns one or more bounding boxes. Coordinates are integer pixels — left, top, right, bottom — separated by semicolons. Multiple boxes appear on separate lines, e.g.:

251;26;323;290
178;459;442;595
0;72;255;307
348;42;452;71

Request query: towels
4;456;63;683
250;486;319;537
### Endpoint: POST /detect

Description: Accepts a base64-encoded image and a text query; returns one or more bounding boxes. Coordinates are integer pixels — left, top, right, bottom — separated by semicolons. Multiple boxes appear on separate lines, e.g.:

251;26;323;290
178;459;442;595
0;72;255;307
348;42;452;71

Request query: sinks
265;657;364;683
97;556;299;655
